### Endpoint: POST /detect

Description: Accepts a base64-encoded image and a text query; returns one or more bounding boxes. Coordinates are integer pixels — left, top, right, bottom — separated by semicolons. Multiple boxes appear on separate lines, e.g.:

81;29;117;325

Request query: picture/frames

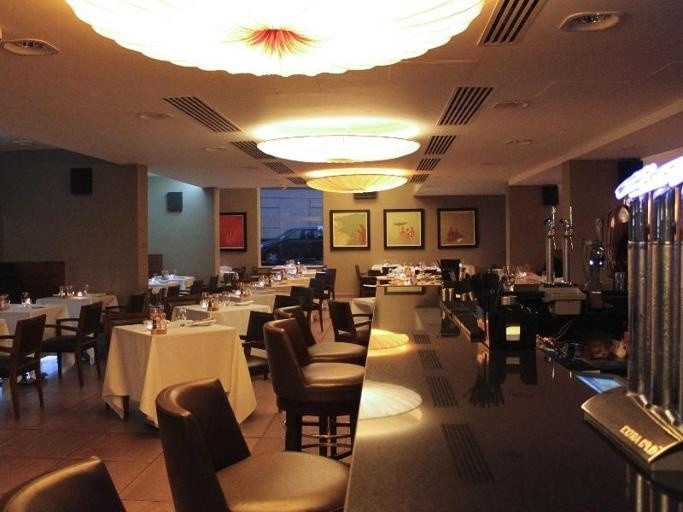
220;212;247;252
384;208;424;249
437;208;477;248
330;209;371;251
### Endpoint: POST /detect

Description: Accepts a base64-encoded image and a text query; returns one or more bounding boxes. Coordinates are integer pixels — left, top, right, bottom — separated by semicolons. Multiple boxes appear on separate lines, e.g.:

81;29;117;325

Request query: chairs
272;302;368;367
154;378;349;511
147;264;336;376
0;287;145;420
353;259;548;293
261;318;367;459
0;455;126;512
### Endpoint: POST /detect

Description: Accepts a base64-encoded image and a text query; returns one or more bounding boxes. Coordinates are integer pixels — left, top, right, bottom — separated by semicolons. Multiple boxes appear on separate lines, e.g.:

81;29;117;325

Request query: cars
261;225;322;265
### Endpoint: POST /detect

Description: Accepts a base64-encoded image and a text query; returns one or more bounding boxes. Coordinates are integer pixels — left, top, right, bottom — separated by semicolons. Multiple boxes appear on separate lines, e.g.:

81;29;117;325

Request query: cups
20;291;28;306
140;305;188;337
199;265;306;308
0;294;9;310
57;283;87;297
150;270;176;283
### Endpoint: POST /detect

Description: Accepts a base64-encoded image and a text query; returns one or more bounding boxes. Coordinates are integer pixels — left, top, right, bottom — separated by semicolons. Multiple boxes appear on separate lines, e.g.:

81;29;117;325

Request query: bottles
440;287;476;303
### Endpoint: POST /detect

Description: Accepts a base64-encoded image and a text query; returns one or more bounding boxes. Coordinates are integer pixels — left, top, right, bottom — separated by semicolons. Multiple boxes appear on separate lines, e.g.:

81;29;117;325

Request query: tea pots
607;338;627;360
